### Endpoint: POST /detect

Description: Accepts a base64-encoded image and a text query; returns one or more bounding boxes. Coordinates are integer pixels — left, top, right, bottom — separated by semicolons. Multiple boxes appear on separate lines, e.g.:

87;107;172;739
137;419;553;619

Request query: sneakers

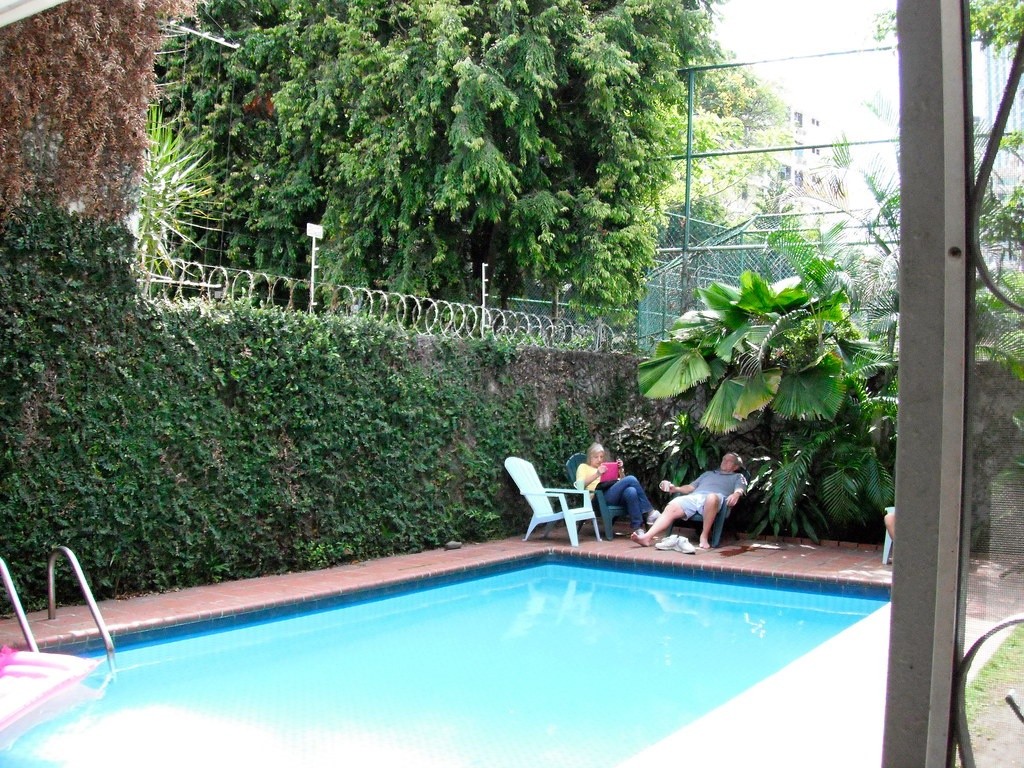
647;510;661;525
655;534;678;550
675;537;696;554
636;528;645;537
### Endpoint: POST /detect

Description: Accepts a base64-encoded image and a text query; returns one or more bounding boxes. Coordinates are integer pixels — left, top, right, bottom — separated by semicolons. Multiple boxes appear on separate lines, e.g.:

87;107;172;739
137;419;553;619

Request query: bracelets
618;468;625;473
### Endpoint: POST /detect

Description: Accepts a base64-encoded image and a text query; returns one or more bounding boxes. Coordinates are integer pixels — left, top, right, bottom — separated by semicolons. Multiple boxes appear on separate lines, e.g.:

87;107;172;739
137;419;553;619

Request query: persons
576;443;662;540
884;513;895;562
631;454;747;549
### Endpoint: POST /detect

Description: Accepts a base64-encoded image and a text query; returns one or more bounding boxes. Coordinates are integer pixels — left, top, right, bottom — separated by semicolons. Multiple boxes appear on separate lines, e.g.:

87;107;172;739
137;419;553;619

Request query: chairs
503;456;602;547
666;465;751;547
566;452;650;541
881;507;895;565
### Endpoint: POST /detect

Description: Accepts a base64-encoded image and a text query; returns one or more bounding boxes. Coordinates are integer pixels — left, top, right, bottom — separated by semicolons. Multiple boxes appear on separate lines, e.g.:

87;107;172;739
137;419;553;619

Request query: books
600;462;620;482
